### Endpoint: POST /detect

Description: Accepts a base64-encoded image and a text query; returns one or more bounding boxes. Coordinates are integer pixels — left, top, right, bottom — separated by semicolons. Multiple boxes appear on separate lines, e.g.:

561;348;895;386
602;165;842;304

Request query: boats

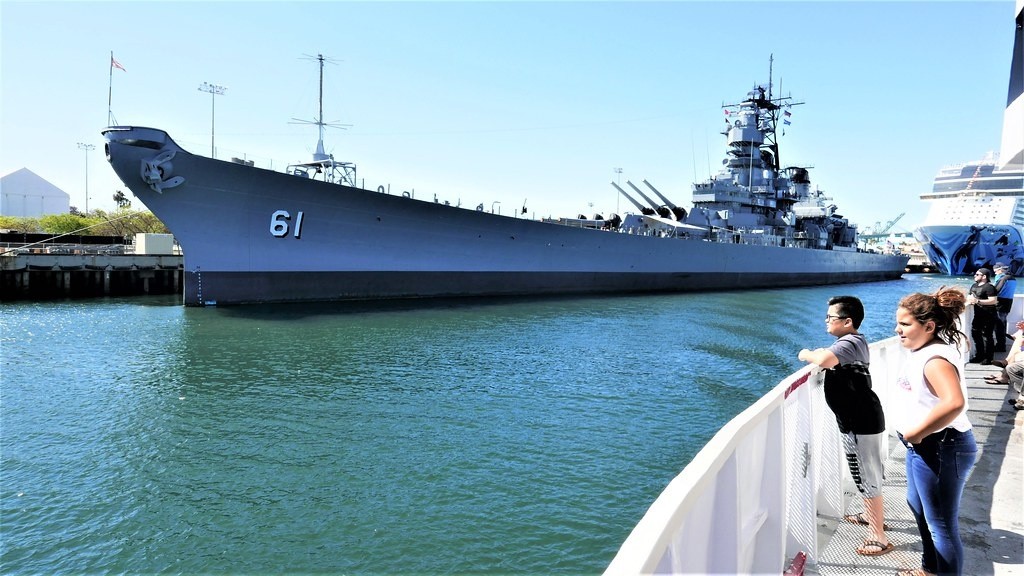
910;0;1024;275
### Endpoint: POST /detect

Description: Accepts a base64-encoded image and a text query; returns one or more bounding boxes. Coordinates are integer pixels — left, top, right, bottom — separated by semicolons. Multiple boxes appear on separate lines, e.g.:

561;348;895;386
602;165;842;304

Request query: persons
965;262;1024;410
894;284;978;576
798;296;894;555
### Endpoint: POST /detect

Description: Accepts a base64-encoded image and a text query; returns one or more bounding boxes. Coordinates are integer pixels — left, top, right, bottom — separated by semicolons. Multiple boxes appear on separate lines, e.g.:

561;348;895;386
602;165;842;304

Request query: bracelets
977;299;980;304
1022;333;1024;335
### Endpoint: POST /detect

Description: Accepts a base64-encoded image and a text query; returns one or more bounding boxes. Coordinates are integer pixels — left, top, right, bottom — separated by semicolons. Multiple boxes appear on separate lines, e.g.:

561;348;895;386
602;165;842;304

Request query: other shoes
1008;396;1024;410
969;356;992;365
990;360;1008;368
993;343;1006;352
1006;333;1015;341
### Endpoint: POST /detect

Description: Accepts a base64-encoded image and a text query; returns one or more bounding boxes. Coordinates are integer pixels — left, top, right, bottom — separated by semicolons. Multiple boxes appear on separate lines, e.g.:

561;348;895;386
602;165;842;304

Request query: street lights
76;142;95;216
198;80;227;158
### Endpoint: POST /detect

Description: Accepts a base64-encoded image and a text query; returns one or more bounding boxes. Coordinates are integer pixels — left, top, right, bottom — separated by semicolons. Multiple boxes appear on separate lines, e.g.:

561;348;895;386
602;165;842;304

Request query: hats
978;268;991;282
993;264;1009;271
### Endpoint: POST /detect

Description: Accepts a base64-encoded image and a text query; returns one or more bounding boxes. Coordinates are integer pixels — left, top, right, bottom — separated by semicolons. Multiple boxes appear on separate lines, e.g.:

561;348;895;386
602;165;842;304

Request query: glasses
975;274;983;276
825;315;847;320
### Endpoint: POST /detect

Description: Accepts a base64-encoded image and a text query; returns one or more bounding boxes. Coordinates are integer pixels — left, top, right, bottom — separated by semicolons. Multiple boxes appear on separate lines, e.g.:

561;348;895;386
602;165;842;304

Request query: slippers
857;541;894;556
984;375;1010;386
843;512;887;529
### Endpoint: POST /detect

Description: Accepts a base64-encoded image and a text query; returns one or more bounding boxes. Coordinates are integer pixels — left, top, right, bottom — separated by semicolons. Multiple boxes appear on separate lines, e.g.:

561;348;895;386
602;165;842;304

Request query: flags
725;118;730;124
110;51;127;72
725;109;730;114
783;101;791;136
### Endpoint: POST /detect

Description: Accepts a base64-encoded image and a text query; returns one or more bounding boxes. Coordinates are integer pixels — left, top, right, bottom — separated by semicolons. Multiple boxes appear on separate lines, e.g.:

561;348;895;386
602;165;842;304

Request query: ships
103;50;912;307
